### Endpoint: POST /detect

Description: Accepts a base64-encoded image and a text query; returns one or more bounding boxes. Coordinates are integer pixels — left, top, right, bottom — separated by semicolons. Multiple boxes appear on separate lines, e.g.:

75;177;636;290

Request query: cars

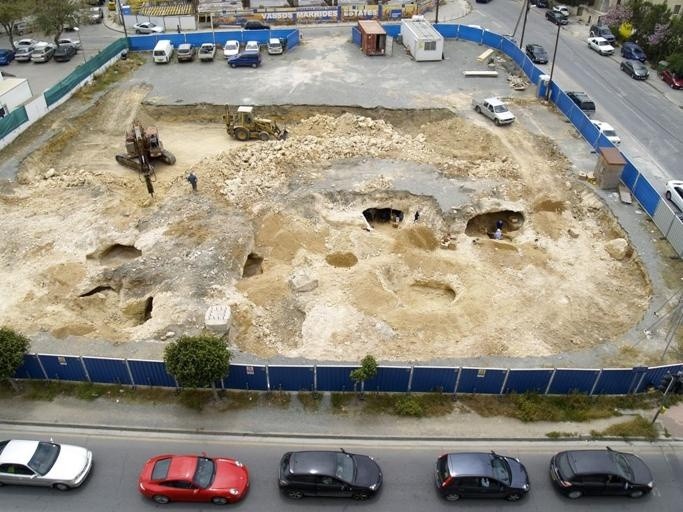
525;44;548;65
138;451;251;507
548;445;652;503
0;38;80;66
531;0;569;26
476;0;492;4
14;1;105;37
665;179;682;213
436;448;530;503
586;24;683;90
133;22;164;34
588;120;621;146
0;436;94;492
151;38;282;71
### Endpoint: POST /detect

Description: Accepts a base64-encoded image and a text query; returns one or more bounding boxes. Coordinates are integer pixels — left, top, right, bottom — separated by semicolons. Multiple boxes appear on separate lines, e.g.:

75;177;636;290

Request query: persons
493;228;502;241
186;172;197;191
496;218;503;229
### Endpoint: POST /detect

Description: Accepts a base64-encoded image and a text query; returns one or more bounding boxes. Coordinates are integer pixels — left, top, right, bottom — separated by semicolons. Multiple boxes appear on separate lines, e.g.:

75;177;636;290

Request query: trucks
471;95;516;128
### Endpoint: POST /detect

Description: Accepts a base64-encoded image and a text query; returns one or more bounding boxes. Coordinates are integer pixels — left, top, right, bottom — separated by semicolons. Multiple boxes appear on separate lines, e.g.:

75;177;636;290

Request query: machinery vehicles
114;117;175;200
222;102;288;142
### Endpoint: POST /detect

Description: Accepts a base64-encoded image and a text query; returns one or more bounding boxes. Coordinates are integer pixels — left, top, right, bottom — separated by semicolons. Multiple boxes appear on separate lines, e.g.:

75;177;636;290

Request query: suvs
243;20;270;30
277;445;384;502
565;89;595;119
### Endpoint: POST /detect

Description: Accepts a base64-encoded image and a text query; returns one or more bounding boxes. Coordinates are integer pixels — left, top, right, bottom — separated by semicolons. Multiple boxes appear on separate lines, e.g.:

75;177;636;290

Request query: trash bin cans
657;60;671;74
